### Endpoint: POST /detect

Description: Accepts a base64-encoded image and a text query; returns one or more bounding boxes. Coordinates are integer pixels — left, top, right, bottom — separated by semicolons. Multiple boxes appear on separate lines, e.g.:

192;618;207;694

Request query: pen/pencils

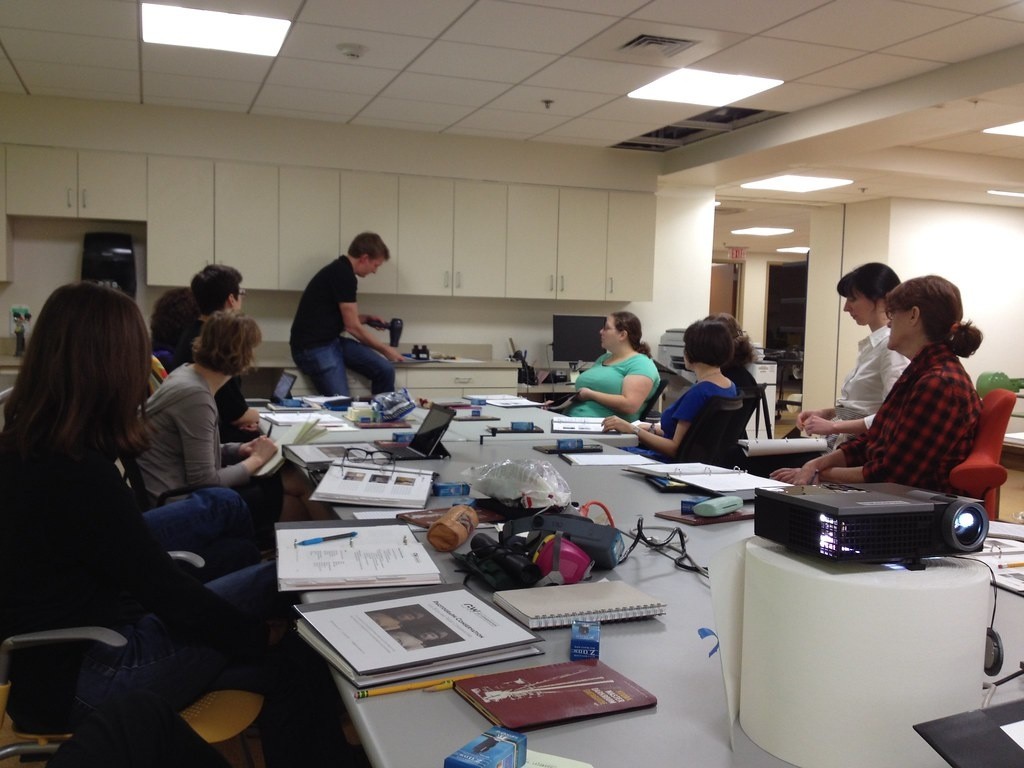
354;674;478;698
297;532;358;545
998;562;1024;568
267;422;273;437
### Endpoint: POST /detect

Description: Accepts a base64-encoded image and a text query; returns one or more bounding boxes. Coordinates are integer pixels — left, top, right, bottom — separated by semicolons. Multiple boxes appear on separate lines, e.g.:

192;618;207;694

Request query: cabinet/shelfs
0;143;657;301
283;368;519;399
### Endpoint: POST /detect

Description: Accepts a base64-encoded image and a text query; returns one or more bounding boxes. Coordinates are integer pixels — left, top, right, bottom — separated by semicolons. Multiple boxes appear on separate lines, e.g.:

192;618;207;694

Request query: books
738;438;828;456
265;413;346;425
493;580;666;629
463;394;546;408
251;419;327;476
551;417;619;434
274;442;446;591
453;658;657;732
292;582;545;688
621;463;794;502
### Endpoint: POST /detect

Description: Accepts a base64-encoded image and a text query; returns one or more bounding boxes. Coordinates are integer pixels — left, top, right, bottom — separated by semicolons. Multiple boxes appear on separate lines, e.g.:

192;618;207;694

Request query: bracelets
649;424;658;433
633;427;641;434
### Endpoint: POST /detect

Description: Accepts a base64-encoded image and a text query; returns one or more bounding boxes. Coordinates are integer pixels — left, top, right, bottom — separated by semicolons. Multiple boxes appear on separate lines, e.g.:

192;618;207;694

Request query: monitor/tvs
553;315;607;383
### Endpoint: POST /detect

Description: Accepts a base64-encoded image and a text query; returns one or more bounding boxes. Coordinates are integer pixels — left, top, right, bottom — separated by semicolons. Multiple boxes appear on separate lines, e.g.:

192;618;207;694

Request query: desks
245;394;1024;768
518;381;577;393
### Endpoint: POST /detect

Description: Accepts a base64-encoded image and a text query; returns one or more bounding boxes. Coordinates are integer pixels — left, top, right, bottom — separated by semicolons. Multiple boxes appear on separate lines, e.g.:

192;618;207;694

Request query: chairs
949;388;1016;521
0;551;265;760
676;390;745;464
639;378;669;422
720;383;768;456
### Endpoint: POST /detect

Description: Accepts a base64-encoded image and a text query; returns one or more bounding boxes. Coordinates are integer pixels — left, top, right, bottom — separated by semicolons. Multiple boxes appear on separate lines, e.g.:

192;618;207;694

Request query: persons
366;610;447;650
0;284;372;768
795;262;909;455
770;274;981;497
291;235;404;397
601;314;757;460
541;310;660;424
138;309;283;563
149;266;261;443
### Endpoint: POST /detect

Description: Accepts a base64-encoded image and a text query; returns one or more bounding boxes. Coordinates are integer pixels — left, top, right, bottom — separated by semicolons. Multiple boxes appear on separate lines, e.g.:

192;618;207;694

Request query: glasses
341;448;397;476
228;287;247;296
885;304;910;320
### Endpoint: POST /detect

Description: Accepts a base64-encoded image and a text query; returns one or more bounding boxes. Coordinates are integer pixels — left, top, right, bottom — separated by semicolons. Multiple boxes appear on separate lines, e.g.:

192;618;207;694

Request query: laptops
246;372;297;407
377;405;457;460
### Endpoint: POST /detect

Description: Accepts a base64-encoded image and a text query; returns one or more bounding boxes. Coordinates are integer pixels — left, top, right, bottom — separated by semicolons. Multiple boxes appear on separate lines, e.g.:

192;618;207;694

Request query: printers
657;328;698;413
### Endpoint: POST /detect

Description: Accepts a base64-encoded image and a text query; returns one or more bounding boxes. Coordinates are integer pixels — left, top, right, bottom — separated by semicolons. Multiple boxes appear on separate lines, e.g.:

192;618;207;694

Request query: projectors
754;482;989;563
